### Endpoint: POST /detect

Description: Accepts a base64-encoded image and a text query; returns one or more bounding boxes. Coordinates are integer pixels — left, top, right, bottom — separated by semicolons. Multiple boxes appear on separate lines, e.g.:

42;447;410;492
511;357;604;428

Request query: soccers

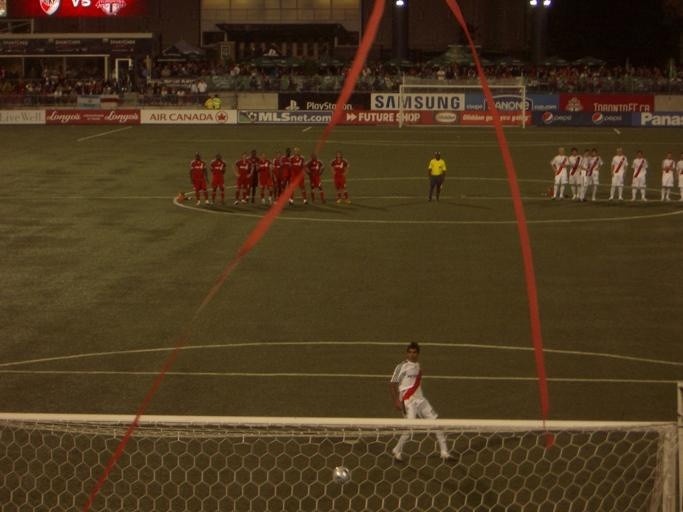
332;466;350;484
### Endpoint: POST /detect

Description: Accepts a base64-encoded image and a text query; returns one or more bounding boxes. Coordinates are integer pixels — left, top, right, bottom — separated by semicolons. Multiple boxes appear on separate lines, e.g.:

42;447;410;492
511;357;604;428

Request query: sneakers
440;453;453;460
392;448;402;461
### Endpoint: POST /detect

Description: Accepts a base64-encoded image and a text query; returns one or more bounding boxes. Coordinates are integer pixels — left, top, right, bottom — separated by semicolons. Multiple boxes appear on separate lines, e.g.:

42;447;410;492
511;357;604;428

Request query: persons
390;341;456;465
427;151;446;202
1;40;681;115
550;146;683;203
189;147;352;207
